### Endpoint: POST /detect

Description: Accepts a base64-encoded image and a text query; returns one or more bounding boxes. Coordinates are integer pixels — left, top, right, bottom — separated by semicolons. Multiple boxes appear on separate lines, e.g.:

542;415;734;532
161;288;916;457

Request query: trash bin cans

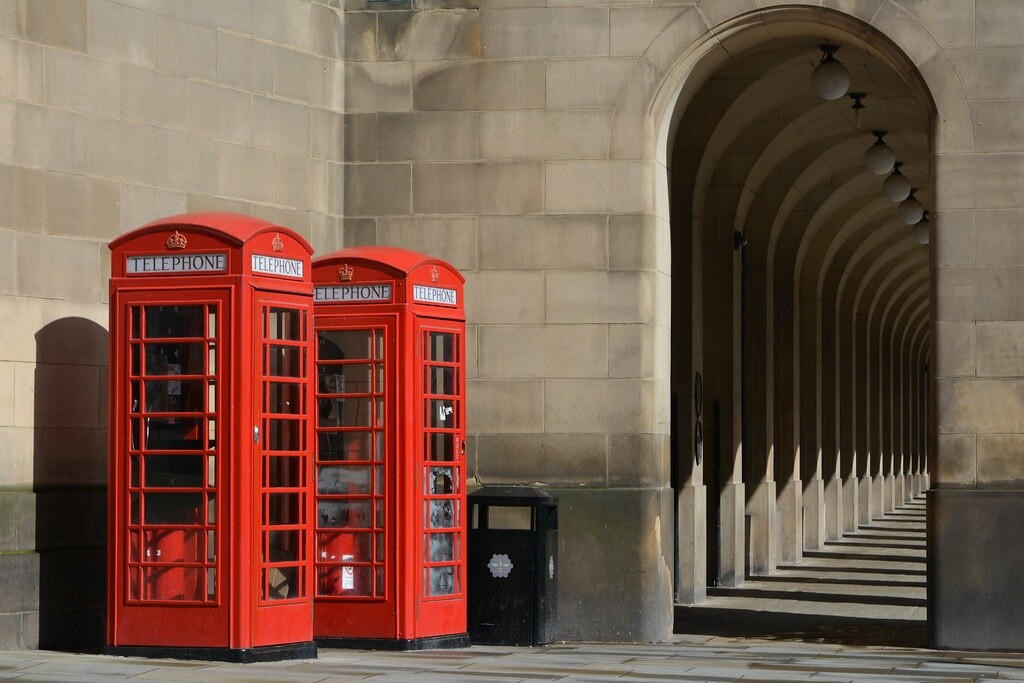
464;486;561;646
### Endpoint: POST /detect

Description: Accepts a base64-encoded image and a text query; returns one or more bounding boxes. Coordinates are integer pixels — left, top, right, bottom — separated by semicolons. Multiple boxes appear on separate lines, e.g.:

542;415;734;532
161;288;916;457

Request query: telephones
313;369;347;418
127;345;187;429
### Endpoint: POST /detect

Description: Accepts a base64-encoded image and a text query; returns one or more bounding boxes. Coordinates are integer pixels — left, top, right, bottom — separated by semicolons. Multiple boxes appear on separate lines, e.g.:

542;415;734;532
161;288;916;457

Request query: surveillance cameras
734;231;747;250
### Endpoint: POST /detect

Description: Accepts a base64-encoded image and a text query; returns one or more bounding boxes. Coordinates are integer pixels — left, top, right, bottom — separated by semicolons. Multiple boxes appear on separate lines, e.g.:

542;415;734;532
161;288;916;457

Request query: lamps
912;210;929;245
898;188;923;225
849;92;867;129
863;131;895;175
812;45;851;100
883;162;911;202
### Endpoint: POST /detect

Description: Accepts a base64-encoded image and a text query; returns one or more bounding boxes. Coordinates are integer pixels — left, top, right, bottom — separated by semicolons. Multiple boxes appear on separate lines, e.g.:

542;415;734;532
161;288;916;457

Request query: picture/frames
694;372;703;418
694;420;703;466
